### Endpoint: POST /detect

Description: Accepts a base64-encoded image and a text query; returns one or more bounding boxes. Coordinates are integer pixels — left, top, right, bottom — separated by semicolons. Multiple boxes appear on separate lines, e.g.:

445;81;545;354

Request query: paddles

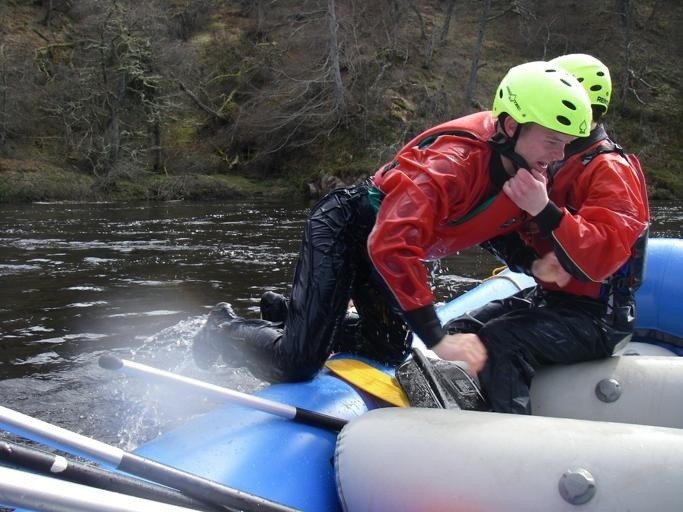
396;345;492;412
325;359;411;408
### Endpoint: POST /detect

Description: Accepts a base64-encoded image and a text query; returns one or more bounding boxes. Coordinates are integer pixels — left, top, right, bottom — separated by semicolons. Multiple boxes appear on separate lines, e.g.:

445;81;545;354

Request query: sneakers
260;291;286;322
192;301;238;370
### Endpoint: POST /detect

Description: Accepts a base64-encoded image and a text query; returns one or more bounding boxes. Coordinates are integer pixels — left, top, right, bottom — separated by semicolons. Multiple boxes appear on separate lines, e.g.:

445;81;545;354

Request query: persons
191;59;594;386
395;52;652;416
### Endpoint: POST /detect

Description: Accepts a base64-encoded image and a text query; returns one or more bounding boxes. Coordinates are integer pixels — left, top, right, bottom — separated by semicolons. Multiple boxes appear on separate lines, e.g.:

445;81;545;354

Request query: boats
0;237;682;510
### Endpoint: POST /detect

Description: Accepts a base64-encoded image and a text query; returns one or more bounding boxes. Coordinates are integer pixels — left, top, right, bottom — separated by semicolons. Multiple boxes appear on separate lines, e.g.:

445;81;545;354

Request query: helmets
550;54;613;117
491;61;593;138
